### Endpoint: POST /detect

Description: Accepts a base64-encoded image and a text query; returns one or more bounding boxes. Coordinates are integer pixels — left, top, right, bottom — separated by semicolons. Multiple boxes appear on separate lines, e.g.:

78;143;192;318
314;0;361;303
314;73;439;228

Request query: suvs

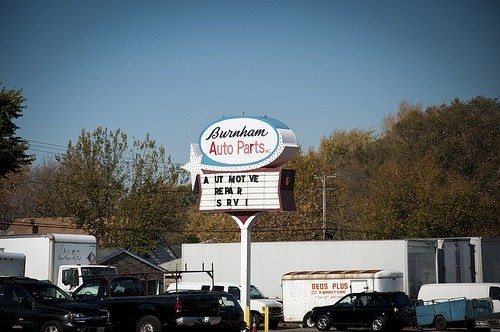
311;291;417;332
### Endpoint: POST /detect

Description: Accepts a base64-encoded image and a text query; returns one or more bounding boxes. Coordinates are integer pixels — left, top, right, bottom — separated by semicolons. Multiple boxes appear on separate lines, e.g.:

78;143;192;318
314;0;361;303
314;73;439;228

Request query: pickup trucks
69;275;221;332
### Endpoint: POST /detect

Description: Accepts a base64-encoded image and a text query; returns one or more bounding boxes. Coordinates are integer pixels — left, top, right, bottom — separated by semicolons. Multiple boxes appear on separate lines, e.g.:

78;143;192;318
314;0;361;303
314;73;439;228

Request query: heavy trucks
0;233;118;295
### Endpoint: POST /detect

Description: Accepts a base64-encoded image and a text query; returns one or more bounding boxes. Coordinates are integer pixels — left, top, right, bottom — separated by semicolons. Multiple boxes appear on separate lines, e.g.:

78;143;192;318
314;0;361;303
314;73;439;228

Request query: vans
417;282;500;316
167;283;283;329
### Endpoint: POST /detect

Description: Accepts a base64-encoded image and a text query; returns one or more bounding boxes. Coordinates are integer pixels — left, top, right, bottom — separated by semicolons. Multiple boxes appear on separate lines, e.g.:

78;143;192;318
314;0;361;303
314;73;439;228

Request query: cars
163;292;246;332
0;276;111;332
